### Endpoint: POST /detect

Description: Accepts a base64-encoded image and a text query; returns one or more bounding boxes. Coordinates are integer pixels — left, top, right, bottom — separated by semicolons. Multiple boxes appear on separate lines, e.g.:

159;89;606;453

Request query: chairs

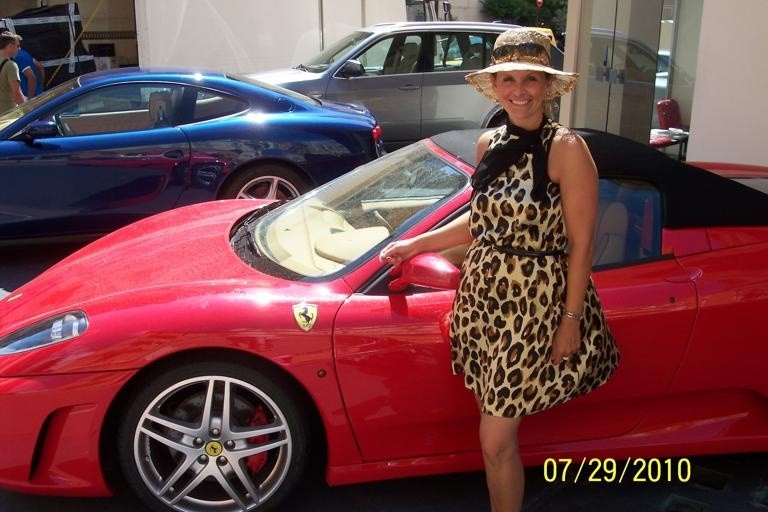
383;43;420;75
591;199;628;267
657;99;689;157
460;42;494;69
147;87;181;129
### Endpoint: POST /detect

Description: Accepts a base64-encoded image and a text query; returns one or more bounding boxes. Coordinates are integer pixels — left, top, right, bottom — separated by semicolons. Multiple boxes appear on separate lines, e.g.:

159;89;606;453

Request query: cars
0;65;383;258
585;27;693;131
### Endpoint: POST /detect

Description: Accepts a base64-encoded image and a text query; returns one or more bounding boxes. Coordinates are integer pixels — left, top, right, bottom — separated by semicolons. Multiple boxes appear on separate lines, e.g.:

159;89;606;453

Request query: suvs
228;18;543;156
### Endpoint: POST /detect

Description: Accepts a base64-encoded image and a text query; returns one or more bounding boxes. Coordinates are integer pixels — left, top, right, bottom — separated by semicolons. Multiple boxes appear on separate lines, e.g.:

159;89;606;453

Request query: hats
465;29;579;103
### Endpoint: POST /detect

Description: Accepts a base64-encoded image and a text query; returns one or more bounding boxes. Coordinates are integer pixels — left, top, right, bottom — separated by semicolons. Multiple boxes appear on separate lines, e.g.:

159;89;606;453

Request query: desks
648;125;687;162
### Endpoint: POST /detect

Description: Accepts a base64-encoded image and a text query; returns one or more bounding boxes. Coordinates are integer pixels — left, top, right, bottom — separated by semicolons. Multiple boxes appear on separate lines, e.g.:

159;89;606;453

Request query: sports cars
1;126;766;512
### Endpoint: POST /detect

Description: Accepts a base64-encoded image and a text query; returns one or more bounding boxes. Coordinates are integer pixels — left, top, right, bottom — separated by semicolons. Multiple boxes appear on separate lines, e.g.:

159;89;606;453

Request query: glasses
492;43;552;63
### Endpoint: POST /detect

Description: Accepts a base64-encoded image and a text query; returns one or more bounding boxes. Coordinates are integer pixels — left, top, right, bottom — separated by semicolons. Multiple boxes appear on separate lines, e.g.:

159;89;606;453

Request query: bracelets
561;308;585;321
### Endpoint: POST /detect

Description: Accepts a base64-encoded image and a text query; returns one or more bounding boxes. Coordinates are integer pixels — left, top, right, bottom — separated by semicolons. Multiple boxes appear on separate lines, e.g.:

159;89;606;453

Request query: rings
562;356;569;360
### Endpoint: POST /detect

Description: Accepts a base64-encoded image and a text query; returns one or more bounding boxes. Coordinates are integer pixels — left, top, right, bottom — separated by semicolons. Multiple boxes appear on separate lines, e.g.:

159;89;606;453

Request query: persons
0;30;32;124
0;26;46;100
378;28;620;512
